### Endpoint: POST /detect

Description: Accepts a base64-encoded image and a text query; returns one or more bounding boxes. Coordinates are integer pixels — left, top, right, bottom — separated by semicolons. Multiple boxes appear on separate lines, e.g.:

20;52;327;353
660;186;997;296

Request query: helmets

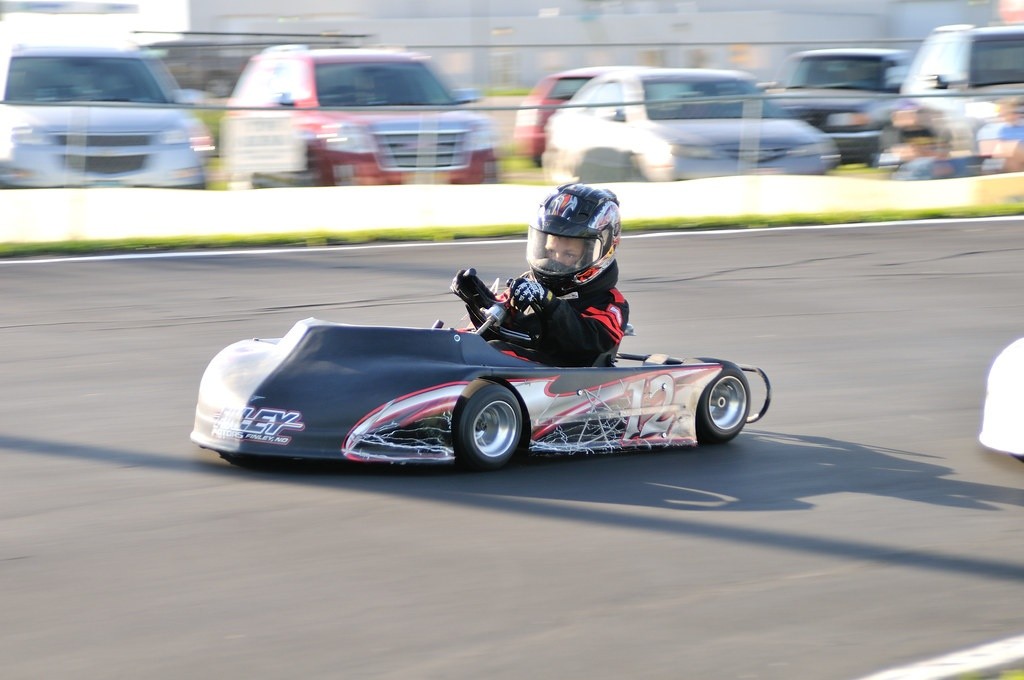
525;181;624;292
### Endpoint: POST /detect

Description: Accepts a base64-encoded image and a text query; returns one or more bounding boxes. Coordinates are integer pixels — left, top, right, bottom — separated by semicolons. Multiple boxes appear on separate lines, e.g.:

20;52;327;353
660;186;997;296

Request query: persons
450;183;629;367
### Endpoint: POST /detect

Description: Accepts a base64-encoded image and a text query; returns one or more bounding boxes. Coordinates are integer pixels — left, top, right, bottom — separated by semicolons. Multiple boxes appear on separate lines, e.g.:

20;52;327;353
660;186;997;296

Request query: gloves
509;275;547;313
450;270;483;302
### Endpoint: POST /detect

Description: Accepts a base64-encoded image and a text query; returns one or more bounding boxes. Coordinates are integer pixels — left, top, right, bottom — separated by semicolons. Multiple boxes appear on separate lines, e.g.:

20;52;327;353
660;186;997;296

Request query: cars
540;74;841;180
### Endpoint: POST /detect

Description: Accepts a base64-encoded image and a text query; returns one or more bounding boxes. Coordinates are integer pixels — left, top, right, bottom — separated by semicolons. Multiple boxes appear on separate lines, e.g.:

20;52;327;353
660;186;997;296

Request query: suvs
217;43;504;187
896;24;1024;180
0;20;208;190
522;72;596;162
767;49;910;166
